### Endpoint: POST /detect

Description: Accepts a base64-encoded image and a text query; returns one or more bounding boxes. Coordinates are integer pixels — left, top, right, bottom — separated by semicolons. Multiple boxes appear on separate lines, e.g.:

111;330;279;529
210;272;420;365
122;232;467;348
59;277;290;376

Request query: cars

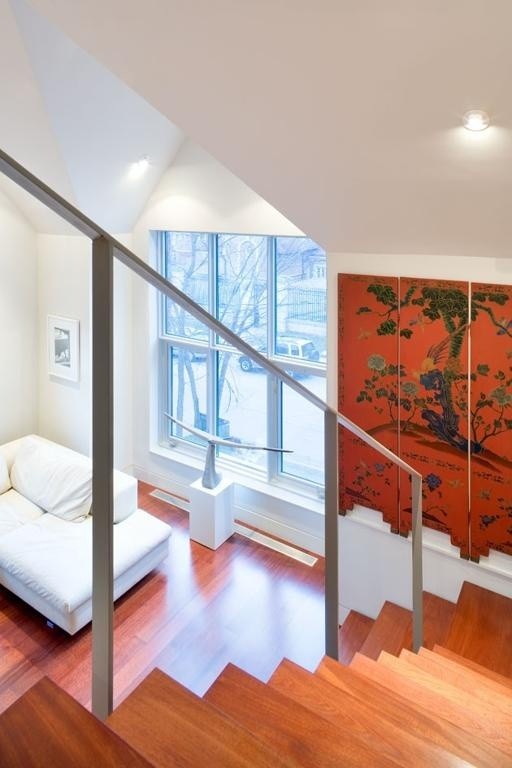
171;326;209;363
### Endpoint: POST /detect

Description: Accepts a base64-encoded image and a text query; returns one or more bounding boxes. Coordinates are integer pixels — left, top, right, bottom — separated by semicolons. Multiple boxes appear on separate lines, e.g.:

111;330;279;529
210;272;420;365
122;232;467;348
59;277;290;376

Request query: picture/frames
48;315;80;384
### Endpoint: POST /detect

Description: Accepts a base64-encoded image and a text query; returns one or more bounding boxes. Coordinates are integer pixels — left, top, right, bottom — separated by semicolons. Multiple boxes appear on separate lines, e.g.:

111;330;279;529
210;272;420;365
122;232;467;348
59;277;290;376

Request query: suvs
237;335;320;380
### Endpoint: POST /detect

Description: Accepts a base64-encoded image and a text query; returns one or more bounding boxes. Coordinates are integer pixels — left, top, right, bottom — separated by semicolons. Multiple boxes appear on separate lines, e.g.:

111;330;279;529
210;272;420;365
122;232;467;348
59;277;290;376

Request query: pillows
0;455;12;495
9;442;93;524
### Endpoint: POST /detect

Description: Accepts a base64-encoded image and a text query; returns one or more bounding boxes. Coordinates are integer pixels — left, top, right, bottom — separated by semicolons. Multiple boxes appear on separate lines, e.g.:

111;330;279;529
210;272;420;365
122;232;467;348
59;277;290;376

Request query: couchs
0;433;172;637
